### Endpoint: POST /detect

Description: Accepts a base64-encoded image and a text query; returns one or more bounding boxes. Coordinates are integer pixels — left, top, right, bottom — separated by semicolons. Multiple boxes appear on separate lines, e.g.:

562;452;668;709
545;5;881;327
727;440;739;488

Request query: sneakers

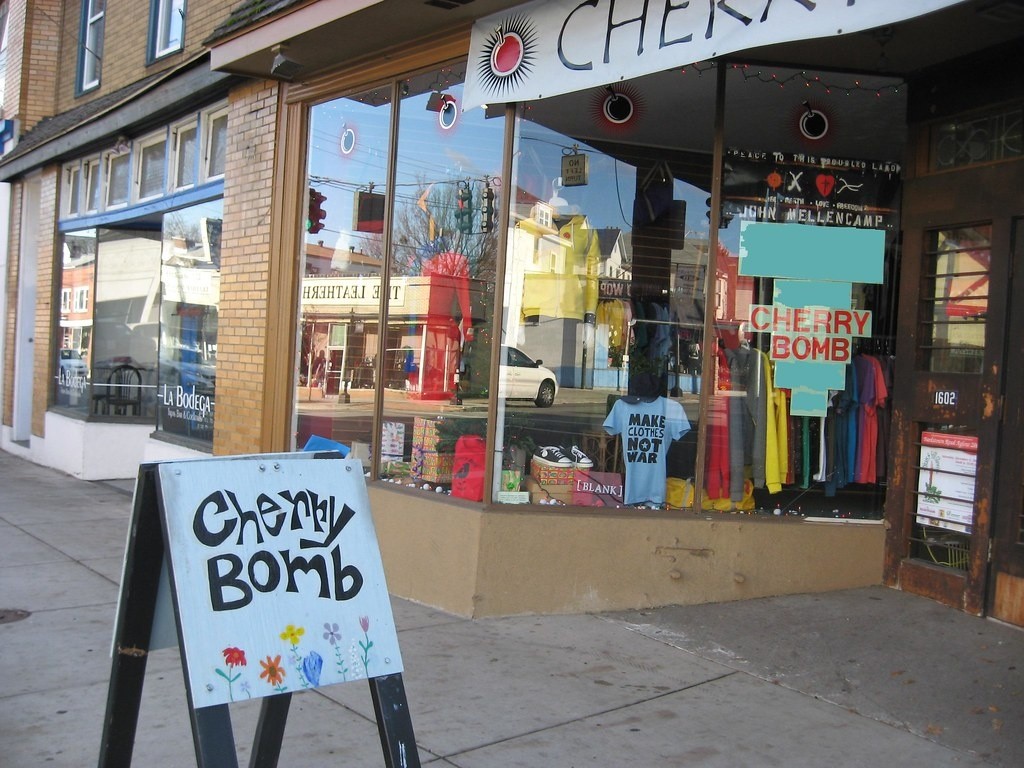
559;445;593;467
532;445;573;468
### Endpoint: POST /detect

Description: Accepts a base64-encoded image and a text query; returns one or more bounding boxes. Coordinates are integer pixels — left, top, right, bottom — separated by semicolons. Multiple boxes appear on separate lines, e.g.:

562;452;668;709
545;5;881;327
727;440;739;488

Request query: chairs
103;365;143;415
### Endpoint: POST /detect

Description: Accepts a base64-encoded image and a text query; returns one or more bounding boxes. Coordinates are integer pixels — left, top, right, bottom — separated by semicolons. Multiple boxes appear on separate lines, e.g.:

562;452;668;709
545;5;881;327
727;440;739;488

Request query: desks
92;395;128;413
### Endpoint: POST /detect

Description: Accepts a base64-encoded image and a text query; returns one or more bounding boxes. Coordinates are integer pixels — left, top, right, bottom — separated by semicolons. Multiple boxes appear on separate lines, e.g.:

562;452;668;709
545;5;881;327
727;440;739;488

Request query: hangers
853;338;893;358
713;327;752;350
638;389;658;402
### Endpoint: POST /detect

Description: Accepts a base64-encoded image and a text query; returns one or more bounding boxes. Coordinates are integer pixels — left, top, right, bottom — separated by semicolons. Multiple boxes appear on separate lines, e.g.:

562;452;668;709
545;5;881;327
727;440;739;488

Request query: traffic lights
308;188;326;234
454;188;474;234
705;197;742;230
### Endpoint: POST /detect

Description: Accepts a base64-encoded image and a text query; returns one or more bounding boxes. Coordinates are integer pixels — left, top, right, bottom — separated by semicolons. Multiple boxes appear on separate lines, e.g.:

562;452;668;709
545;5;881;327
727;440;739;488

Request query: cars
59;347;90;383
500;343;559;409
160;344;215;397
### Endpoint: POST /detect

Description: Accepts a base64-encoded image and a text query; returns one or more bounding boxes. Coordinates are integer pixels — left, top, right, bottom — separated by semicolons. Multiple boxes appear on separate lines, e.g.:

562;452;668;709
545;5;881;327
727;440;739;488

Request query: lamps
270;45;304;80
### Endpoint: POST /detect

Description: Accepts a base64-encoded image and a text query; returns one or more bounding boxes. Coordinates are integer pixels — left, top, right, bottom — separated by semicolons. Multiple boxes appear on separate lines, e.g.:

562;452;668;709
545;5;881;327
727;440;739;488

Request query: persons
404;235;473;401
300;350;325;387
